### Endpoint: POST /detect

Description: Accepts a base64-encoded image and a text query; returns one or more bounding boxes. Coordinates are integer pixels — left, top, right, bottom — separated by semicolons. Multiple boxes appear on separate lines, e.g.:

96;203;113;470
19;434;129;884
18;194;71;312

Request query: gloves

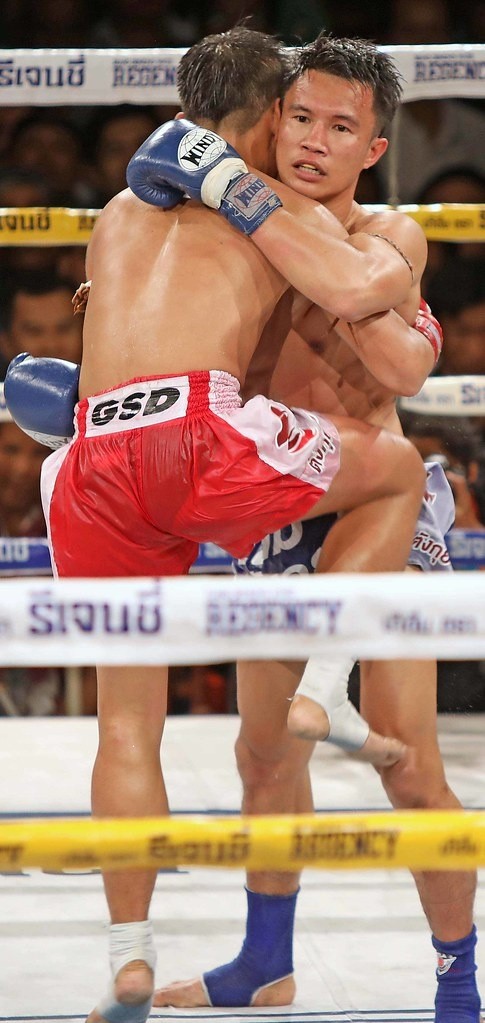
411;294;445;364
3;351;81;451
126;118;284;236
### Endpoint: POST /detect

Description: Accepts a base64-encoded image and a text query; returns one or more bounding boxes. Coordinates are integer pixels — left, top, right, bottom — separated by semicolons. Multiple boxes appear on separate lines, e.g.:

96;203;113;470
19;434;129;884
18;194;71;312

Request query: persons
39;30;442;1023
0;0;485;720
4;34;481;1023
